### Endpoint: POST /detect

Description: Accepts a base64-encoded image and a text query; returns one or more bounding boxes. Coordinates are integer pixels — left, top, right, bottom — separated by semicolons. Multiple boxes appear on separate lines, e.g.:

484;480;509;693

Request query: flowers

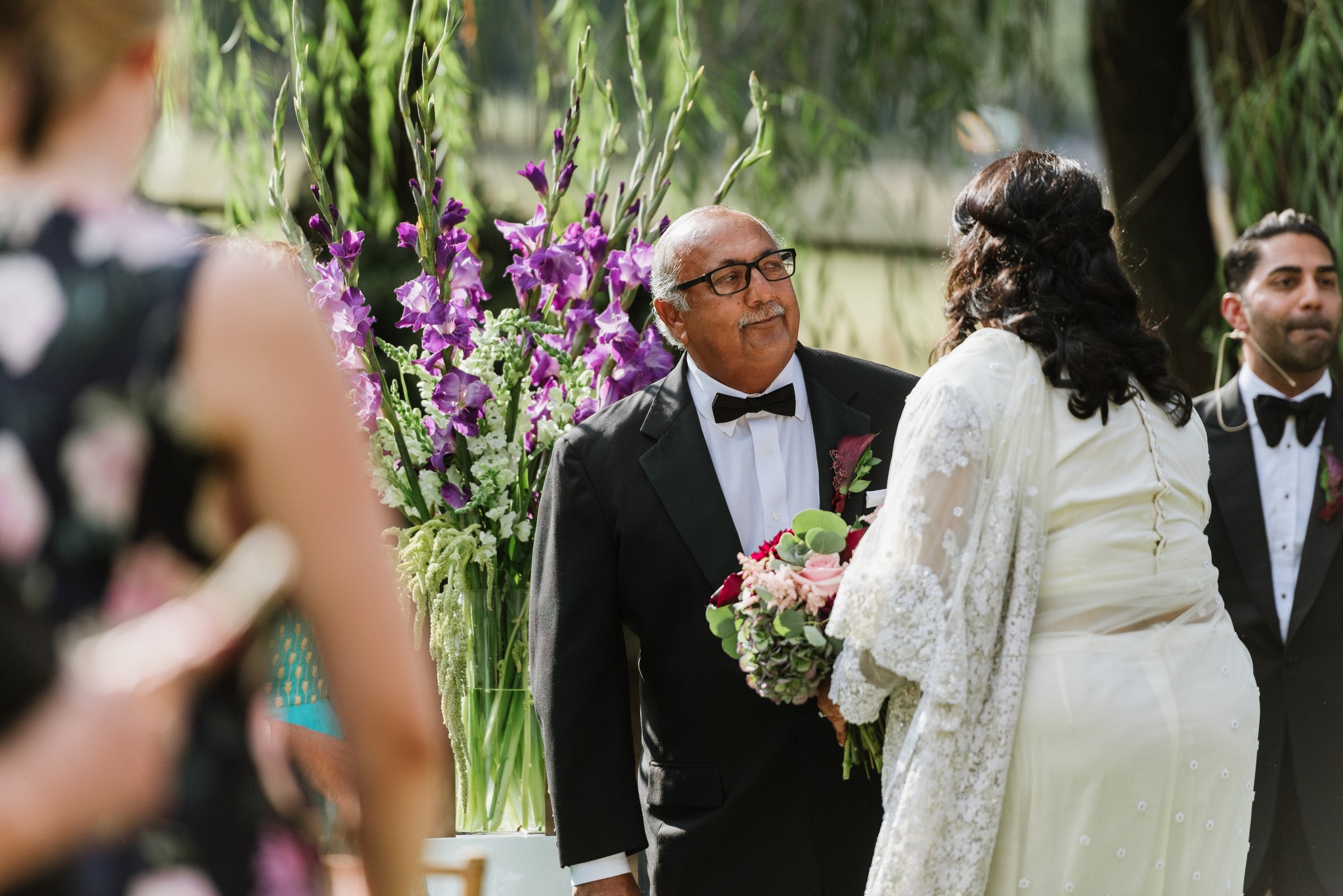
194;0;772;680
1313;443;1343;532
697;504;908;781
819;429;886;516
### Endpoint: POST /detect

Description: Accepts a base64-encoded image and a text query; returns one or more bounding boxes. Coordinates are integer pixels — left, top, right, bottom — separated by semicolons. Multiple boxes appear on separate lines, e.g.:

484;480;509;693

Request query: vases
428;580;556;836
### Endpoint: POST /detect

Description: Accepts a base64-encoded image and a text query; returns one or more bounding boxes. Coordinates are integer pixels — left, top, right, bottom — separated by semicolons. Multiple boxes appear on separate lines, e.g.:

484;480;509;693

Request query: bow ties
1253;390;1333;447
711;383;797;423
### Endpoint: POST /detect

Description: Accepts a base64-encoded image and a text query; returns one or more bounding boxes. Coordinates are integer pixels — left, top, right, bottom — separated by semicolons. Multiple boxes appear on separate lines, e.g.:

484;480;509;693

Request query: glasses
671;245;796;297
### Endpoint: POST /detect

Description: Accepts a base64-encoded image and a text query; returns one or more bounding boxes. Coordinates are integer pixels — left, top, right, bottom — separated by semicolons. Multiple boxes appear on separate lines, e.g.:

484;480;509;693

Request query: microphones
1232;328;1298;389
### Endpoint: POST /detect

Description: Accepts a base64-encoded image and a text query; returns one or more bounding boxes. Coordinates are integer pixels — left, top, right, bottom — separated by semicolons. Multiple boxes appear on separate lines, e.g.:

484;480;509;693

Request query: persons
821;149;1258;896
2;620;189;882
1174;210;1343;896
2;0;451;896
523;208;921;896
